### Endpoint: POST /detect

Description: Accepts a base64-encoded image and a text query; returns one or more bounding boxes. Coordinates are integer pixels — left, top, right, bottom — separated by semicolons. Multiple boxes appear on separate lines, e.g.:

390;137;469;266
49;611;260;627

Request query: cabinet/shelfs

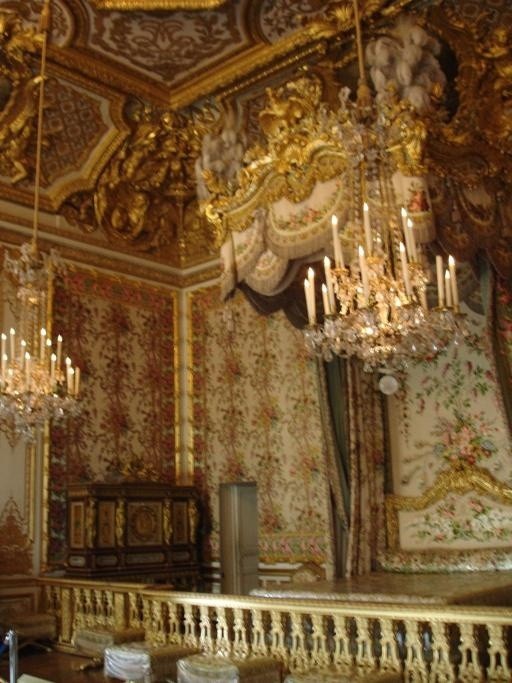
65;477;205;599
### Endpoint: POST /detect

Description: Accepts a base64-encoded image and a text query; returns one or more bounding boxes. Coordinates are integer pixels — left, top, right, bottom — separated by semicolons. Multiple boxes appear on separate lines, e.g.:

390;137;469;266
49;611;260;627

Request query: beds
249;466;511;602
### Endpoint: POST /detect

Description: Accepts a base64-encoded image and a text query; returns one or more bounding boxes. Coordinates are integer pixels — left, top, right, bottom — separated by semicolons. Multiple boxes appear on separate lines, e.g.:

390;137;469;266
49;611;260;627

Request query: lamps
296;0;466;395
1;0;85;451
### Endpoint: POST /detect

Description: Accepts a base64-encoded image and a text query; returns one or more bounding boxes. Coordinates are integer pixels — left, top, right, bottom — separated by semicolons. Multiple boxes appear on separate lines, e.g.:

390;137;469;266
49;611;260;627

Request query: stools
2;615;58;660
71;626;145;672
175;653;282;682
103;640;198;683
